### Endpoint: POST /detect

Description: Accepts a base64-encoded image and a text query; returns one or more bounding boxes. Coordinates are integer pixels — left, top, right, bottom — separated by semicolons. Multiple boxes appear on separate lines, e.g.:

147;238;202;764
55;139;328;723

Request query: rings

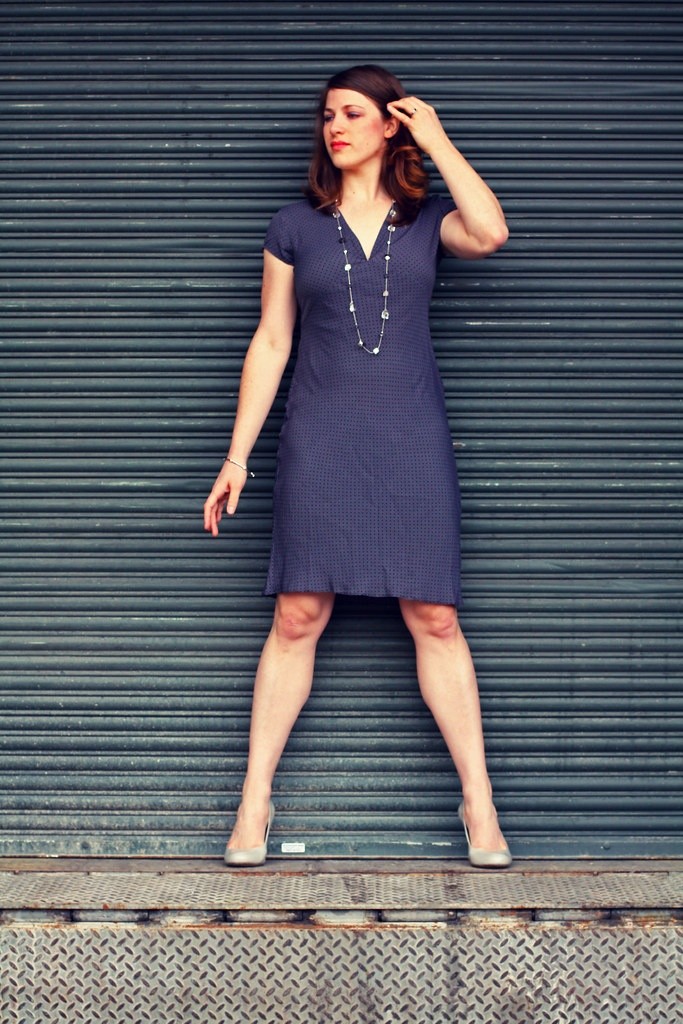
411;109;416;115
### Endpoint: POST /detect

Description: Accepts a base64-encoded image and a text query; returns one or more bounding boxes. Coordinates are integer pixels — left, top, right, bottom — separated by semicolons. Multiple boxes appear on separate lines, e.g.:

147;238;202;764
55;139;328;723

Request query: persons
204;65;515;867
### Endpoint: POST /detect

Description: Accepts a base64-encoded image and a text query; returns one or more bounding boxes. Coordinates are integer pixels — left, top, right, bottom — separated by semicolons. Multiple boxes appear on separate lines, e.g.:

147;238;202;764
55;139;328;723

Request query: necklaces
333;199;397;354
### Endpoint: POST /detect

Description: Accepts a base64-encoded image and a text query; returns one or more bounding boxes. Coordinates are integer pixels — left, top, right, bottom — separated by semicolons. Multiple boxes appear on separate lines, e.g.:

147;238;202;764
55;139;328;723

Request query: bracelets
224;457;255;478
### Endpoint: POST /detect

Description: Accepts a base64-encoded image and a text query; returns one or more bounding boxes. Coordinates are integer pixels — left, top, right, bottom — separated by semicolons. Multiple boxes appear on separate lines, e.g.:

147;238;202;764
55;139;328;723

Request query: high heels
456;799;513;867
224;798;275;867
